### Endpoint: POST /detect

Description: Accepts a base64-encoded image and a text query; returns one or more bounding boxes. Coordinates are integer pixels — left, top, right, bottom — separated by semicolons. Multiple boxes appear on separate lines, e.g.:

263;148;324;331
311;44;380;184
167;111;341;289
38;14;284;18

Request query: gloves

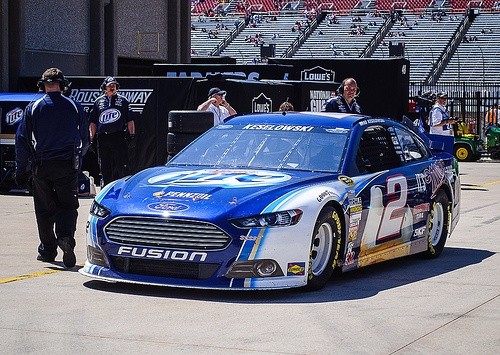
128;134;136;149
88;138;96;153
15;165;30;189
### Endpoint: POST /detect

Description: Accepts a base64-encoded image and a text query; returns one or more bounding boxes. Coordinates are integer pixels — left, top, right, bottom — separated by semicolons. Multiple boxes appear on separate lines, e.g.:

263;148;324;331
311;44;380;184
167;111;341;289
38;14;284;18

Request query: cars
77;109;462;292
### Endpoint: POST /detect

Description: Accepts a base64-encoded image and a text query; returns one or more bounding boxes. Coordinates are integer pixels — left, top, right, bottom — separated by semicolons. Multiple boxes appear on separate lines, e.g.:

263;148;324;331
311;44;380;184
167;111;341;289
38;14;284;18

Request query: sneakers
37;254;55;260
59;239;76;268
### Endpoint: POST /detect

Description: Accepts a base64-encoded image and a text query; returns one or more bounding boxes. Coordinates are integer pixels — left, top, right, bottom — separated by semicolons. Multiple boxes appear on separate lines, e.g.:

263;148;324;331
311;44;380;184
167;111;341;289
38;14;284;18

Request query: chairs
189;0;500;13
356;131;401;173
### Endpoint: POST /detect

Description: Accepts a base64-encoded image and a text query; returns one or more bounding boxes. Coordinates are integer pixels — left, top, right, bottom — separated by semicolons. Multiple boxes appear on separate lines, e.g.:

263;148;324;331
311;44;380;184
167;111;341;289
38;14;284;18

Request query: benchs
191;12;500;87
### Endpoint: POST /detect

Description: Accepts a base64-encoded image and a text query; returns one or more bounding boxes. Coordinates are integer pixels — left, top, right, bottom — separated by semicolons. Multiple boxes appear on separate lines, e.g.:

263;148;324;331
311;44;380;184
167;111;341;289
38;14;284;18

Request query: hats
208;88;225;96
437;91;447;98
104;76;118;86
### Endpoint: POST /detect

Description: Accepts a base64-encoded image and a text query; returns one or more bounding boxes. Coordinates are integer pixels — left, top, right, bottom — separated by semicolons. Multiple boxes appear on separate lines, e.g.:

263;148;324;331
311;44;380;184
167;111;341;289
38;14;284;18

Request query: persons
197;85;238;127
90;77;138;200
191;2;500;124
14;65;91;269
317;78;361;118
426;91;457;163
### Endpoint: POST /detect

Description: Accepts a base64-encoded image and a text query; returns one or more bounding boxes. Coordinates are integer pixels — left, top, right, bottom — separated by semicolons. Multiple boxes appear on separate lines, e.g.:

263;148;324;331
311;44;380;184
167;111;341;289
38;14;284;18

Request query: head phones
37;79;69;88
100;77;120;91
337;81;360;96
